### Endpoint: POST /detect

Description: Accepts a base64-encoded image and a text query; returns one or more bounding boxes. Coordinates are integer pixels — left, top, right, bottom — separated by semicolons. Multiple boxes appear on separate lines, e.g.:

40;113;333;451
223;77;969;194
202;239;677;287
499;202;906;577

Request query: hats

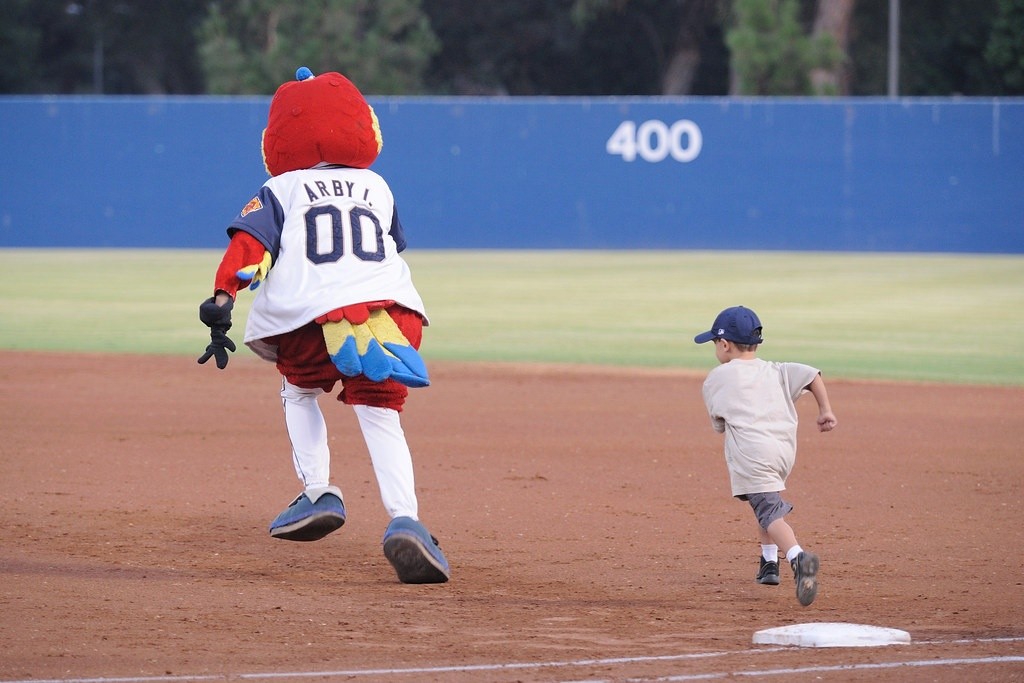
694;305;762;344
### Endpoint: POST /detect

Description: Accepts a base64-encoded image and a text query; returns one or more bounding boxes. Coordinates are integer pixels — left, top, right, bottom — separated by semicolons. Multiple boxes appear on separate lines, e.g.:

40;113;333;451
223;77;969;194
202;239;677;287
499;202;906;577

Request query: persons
695;306;837;605
199;66;447;582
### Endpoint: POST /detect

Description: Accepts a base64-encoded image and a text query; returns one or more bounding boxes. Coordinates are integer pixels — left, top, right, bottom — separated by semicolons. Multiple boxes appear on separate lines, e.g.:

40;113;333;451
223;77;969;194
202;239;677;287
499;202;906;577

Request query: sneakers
755;549;786;585
790;550;819;605
